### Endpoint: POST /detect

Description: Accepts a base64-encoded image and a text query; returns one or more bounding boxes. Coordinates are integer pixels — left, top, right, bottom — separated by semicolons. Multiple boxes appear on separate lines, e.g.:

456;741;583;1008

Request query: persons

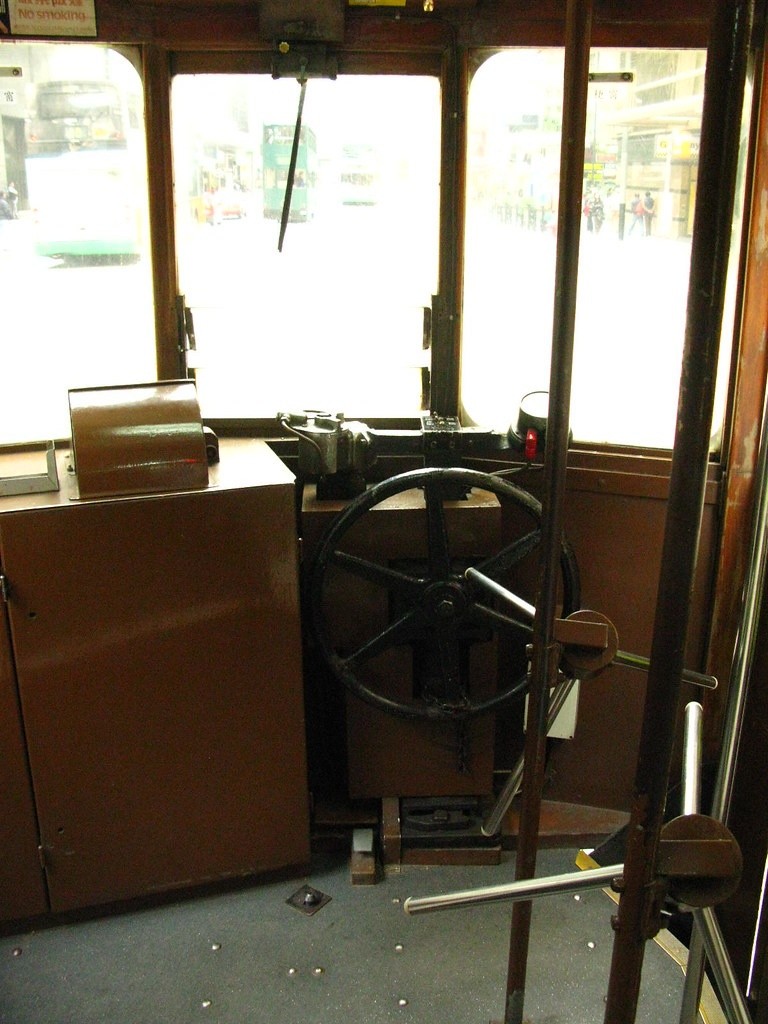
0;182;18;220
631;191;655;236
583;193;603;232
